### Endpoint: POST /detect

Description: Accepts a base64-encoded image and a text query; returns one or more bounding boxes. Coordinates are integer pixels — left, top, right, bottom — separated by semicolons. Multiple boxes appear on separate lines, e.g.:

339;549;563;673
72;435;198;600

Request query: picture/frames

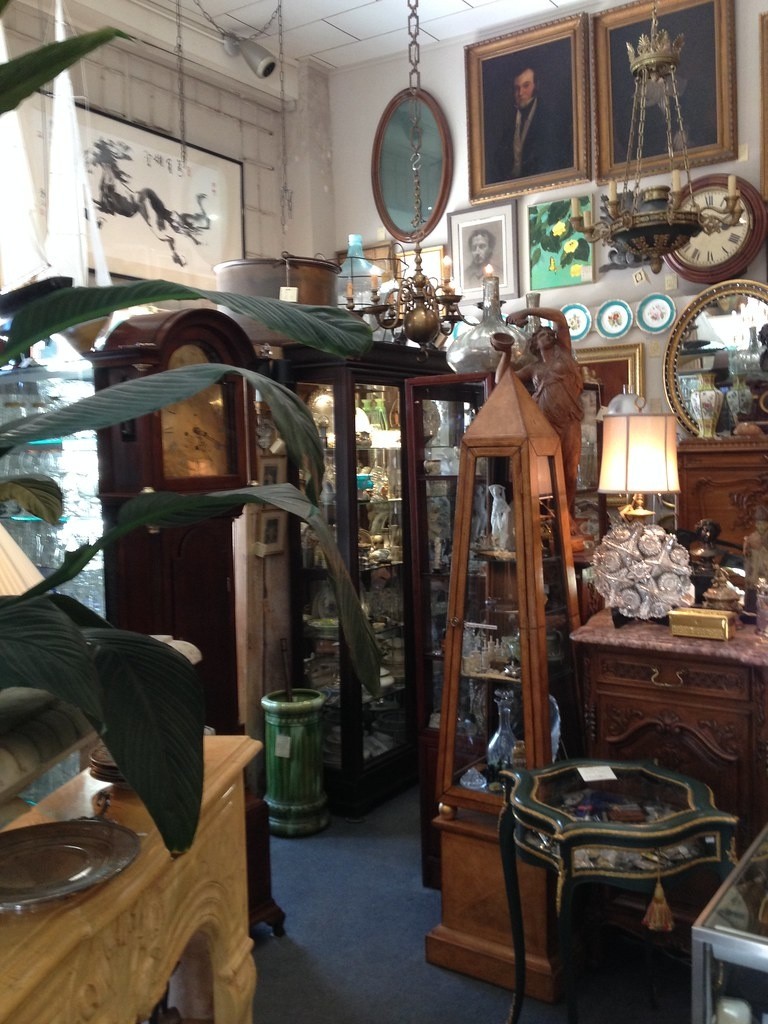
20;86;245;292
528;192;595;292
335;239;397;307
396;246;447;320
589;0;737;187
464;11;592;208
576;343;645;506
447;199;520;306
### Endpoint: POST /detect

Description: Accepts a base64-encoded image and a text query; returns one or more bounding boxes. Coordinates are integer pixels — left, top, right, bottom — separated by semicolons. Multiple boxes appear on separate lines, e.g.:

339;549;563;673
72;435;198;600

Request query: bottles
336;233;401;343
725;373;754;427
689;372;724;440
520;292;545;332
446;277;533;374
486;698;527;771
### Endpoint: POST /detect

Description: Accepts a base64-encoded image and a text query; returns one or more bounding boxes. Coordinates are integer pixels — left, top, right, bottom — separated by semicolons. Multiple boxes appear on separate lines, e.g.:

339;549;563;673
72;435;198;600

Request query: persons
489;484;511;548
582;391;596;423
473;485;487;539
743;532;768;612
495;307;585;535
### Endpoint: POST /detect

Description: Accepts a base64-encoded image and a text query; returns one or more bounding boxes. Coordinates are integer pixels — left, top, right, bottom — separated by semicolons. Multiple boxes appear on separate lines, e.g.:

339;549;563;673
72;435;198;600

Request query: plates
453;302;593;342
89;740;135;790
0;820;143;914
634;292;677;335
594;299;634;340
306;586;339;634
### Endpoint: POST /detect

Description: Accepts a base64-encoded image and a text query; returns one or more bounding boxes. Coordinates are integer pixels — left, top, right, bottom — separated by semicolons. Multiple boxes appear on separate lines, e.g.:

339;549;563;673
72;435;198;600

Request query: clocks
85;308;289;949
661;172;768;284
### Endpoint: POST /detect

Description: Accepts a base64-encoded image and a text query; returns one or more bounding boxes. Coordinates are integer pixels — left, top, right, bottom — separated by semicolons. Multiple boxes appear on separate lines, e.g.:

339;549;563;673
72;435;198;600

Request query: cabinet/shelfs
290;340;482;822
691;825;768;1024
573;600;768;953
400;370;609;900
425;373;583;1007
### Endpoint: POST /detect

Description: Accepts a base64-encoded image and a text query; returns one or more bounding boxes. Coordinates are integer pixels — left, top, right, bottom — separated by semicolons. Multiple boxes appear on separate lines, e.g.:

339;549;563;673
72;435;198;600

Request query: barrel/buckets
209;250;342;343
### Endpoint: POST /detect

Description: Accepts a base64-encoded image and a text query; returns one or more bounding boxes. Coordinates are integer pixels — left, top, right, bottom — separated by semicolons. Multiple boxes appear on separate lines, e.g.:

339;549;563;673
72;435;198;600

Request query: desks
482;756;739;1024
0;729;267;1024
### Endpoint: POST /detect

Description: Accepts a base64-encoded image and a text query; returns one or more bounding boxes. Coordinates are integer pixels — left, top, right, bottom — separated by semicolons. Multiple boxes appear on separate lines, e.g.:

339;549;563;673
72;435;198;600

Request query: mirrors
661;277;768;439
372;87;456;246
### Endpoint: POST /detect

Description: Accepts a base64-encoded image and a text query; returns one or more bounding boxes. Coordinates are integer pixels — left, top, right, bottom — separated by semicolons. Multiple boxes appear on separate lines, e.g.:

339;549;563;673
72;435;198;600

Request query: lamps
341;0;507;362
223;35;278;79
570;0;745;274
595;412;680;523
